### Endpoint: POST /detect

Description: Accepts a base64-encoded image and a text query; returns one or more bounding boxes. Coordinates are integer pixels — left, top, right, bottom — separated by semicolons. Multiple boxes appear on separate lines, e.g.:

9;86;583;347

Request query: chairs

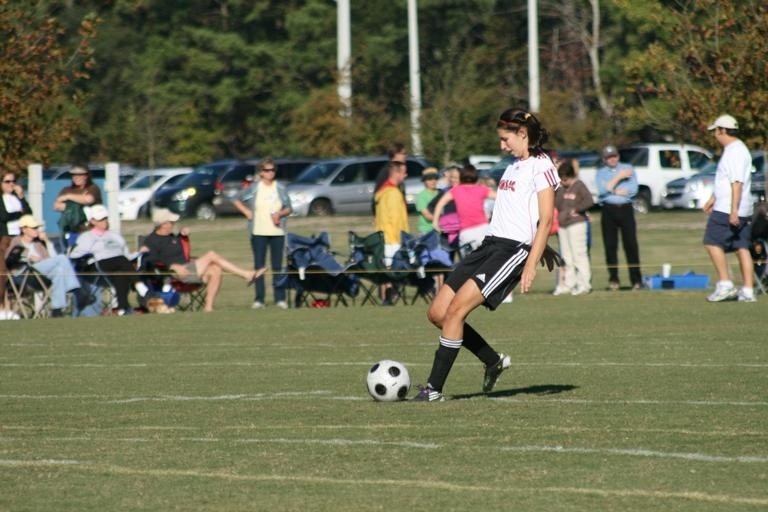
0;244;66;319
140;234;212;314
65;231;149;316
344;231;409;307
748;204;767;294
397;231;456;306
272;230;353;309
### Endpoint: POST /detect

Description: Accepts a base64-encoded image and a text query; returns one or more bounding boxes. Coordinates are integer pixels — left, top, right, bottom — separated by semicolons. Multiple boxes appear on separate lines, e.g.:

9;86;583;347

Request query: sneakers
5;311;20;320
482;352;512;392
410;384;445;402
251;302;264;309
735;288;757;303
706;280;737;302
276;300;289;310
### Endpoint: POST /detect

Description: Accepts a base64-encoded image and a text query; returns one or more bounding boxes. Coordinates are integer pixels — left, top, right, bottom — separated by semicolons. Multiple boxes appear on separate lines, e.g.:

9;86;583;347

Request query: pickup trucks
551;142;714;216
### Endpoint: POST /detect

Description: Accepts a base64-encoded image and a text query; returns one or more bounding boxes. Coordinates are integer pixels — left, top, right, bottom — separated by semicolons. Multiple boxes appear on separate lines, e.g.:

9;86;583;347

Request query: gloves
539;244;565;272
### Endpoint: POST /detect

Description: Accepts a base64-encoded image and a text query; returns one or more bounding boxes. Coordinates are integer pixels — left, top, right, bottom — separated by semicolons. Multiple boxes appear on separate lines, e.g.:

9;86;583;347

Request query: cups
662;263;671;277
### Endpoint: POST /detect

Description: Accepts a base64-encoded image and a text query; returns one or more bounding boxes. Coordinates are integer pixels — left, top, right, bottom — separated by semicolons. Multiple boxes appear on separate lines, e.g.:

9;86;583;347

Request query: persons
701;114;756;303
374;138;594;304
228;156;293;310
142;206;268;313
593;144;644;291
54;161;156;317
410;108;561;403
2;169;96;316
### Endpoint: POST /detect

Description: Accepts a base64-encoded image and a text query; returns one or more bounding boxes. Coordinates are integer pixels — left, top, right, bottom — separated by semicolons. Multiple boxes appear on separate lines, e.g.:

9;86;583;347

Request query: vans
286;156;442;218
21;159;318;223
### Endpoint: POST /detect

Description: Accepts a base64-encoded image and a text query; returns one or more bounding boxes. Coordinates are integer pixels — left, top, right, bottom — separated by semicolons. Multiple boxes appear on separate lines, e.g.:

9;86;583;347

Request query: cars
660;150;768;209
466;155;508;173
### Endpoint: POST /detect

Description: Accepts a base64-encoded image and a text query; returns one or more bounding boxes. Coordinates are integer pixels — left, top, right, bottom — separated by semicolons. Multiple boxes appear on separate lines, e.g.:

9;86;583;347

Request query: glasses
262;168;275;172
3;179;16;184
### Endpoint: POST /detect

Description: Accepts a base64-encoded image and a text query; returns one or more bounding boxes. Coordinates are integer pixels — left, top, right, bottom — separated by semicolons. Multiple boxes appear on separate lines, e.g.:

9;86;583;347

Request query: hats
420;166;441;182
18;214;44;228
707;114;739;131
602;145;618;159
68;166;88;174
85;204;109;222
152;208;180;227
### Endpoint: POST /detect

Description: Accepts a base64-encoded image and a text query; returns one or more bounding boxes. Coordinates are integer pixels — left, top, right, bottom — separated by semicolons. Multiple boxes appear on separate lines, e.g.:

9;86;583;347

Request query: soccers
367;360;410;402
